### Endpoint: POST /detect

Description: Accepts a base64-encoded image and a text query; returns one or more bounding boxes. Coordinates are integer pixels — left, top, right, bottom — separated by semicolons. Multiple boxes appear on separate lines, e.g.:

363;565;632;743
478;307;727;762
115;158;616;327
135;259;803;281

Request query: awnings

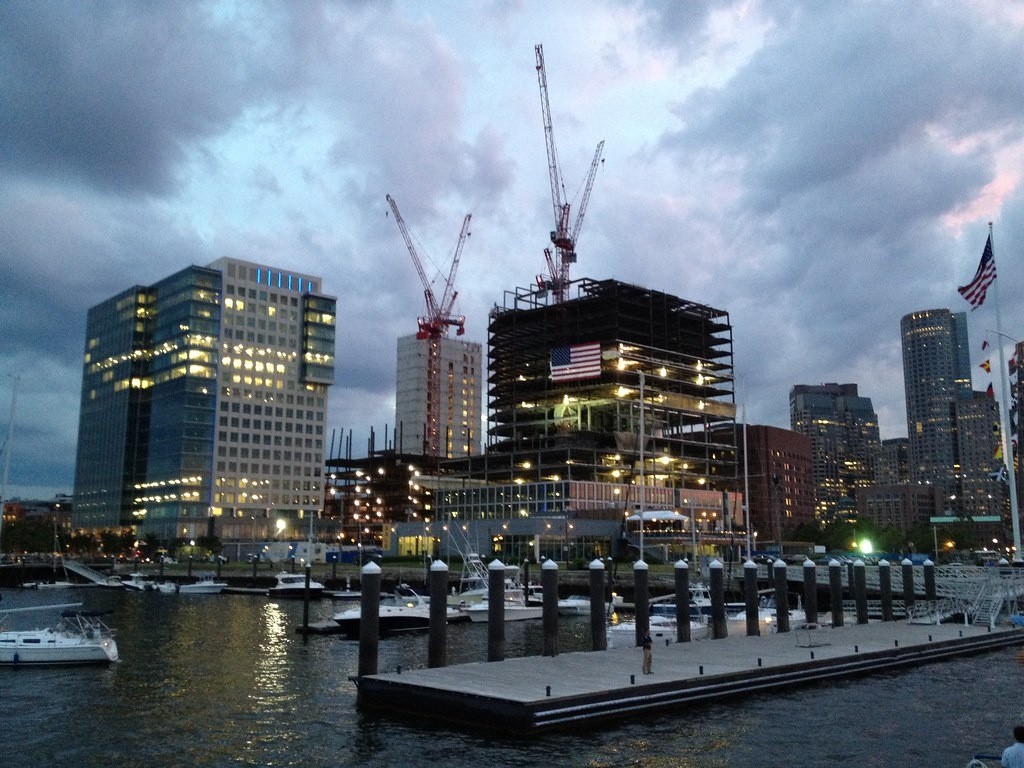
625;509;689;522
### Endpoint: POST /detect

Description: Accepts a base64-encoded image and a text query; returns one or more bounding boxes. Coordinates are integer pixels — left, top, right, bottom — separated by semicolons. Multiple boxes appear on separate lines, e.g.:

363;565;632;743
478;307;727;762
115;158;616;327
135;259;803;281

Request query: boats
177;573;229;593
123;571;178;595
557;595;613;615
96;576;124;586
333;574;469;632
269;561;325;599
0;601;120;664
443;523;545;622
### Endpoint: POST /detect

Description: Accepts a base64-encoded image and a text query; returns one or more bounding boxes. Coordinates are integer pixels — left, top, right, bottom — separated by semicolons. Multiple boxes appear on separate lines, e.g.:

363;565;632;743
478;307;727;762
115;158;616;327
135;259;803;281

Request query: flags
550;341;601;382
958;234;997;310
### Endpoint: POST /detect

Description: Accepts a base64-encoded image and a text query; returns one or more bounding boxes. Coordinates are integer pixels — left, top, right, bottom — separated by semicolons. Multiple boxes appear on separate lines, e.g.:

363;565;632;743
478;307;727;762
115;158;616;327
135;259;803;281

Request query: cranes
386;194;472;465
532;44;605;304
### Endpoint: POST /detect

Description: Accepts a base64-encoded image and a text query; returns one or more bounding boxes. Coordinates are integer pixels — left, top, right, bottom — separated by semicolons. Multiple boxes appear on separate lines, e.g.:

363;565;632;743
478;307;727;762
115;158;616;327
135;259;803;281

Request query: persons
14;556;18;563
1001;724;1024;768
642;629;654;675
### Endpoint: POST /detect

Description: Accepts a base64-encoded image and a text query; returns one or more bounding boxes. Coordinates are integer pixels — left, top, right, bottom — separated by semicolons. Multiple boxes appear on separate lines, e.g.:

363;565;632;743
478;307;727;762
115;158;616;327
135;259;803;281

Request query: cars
754;553;777;564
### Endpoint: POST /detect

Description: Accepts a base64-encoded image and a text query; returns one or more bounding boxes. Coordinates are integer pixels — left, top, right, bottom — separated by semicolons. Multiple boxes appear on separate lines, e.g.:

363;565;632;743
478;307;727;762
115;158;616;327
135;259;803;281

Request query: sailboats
647;499;712;619
716;402;807;634
599;370;710;647
19;514;83;589
